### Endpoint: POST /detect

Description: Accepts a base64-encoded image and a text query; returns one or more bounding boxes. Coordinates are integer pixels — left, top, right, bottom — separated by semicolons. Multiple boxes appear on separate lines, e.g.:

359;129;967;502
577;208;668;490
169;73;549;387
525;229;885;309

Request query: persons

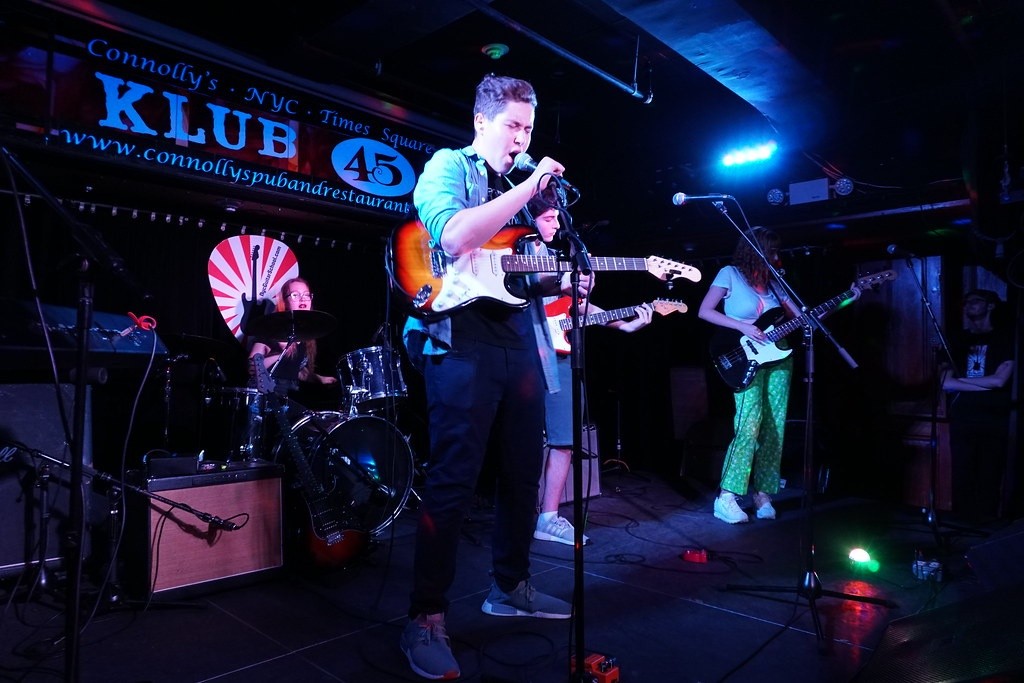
249;279;338;387
699;232;861;525
939;294;1016;524
400;76;595;681
529;188;653;547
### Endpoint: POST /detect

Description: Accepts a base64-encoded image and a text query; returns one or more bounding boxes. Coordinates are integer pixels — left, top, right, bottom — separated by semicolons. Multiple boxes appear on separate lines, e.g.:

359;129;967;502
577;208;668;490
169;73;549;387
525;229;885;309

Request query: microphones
515;152;579;195
215;359;227;382
210;518;239;531
378;484;397;499
372;323;387;343
580;451;596;460
887;245;919;259
673;192;733;205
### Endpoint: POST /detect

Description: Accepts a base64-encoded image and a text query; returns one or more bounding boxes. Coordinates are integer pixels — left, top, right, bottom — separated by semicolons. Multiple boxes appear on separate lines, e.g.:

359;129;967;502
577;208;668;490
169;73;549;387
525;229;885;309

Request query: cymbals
243;308;336;345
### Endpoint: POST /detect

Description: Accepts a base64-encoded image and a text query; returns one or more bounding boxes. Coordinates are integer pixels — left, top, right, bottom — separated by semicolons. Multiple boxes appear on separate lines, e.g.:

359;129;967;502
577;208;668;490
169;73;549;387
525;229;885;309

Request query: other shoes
714;497;747;524
748;494;776;520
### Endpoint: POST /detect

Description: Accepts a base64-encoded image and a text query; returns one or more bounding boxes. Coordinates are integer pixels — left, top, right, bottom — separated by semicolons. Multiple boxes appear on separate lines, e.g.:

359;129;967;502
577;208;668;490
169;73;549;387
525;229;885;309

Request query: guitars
251;351;386;592
708;269;900;393
545;294;687;354
385;212;703;324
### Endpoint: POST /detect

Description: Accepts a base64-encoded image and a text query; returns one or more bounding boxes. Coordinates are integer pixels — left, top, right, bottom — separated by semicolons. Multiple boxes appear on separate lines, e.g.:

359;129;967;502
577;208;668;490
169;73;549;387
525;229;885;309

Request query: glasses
283;291;314;302
967;298;989;304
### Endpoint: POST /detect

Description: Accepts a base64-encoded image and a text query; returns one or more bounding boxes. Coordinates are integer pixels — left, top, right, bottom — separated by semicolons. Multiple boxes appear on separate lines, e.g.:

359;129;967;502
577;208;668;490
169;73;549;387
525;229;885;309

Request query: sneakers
400;620;461;680
481;579;573;619
533;511;590;546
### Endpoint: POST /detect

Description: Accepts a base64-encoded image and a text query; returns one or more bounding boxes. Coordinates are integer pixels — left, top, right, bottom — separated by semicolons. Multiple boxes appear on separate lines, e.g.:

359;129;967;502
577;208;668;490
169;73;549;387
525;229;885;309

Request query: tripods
15;442;221;660
707;199;899;655
863;263;996;583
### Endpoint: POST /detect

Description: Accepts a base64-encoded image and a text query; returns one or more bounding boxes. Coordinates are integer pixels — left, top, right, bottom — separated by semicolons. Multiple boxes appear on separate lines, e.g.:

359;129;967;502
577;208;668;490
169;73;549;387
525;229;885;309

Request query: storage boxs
535;423;601;514
0;383;90;576
122;463;282;595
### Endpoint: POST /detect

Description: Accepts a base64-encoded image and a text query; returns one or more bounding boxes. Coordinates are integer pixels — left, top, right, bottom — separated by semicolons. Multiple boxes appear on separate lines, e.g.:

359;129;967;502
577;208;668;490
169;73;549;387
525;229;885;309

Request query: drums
334;344;410;408
277;410;416;536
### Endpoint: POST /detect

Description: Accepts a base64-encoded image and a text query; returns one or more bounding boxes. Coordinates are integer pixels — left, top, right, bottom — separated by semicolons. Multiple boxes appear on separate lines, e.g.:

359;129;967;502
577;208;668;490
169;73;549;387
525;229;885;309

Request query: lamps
766;177;854;206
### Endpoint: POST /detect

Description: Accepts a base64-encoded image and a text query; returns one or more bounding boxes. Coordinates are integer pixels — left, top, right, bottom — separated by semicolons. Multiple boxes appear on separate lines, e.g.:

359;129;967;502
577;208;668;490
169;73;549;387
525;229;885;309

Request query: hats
965;290;1002;306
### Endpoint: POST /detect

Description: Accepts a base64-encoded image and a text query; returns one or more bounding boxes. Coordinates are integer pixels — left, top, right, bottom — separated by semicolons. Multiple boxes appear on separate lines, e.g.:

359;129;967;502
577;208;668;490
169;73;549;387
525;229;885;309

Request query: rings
758;332;762;335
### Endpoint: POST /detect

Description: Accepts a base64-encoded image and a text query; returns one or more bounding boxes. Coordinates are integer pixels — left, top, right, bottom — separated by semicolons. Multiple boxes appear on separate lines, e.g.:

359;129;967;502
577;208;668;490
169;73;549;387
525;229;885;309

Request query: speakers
963;519;1024;598
125;460;288;603
857;582;1024;683
537;424;600;511
